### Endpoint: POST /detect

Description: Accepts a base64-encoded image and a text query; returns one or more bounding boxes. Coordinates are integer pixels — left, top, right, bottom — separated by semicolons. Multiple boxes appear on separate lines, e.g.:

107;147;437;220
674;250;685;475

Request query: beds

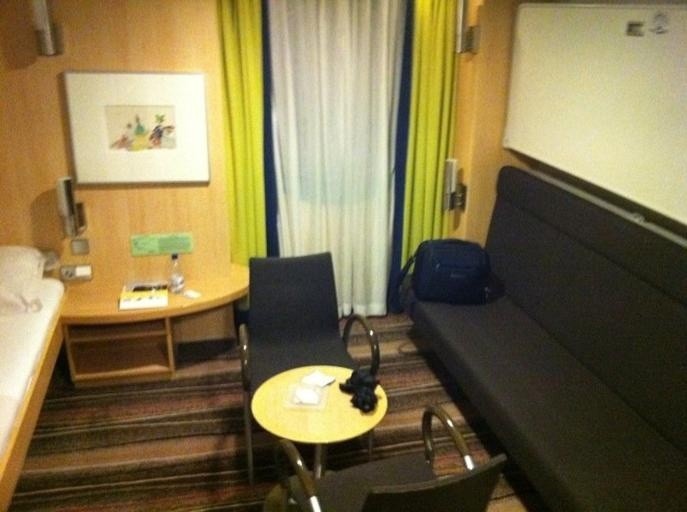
0;239;71;511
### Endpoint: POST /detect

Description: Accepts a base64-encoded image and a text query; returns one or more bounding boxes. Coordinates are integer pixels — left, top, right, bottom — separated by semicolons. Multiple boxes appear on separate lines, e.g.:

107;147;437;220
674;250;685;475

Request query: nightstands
57;261;250;390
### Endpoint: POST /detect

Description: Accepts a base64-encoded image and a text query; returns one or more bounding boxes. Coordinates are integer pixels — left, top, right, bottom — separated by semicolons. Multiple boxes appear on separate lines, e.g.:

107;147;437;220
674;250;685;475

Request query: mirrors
0;244;47;313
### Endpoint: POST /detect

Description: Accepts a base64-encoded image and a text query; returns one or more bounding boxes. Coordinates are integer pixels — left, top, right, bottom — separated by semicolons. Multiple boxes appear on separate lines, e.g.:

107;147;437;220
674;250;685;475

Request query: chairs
266;400;505;511
236;250;381;486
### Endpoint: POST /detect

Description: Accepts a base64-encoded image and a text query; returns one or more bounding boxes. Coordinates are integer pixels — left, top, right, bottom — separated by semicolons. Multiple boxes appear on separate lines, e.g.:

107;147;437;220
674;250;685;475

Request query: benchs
400;164;685;512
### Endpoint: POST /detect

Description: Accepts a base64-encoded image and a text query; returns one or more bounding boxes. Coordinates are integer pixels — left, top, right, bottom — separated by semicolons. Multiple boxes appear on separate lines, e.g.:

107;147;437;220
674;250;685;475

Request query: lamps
30;0;60;57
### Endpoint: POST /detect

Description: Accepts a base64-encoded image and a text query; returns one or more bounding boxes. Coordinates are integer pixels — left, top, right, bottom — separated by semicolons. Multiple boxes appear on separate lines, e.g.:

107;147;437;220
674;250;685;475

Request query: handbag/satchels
387;238;493;315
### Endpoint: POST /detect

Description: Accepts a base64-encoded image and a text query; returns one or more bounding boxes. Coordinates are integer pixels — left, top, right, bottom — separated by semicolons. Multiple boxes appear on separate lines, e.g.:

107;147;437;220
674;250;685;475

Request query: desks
250;363;389;484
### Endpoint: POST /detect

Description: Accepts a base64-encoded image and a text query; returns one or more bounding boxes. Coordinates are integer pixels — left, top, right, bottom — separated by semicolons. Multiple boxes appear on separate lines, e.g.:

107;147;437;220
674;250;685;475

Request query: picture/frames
58;68;214;187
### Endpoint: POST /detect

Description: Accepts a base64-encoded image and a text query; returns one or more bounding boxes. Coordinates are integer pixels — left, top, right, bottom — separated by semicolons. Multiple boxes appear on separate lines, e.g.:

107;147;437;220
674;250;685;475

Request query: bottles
168;254;186;296
